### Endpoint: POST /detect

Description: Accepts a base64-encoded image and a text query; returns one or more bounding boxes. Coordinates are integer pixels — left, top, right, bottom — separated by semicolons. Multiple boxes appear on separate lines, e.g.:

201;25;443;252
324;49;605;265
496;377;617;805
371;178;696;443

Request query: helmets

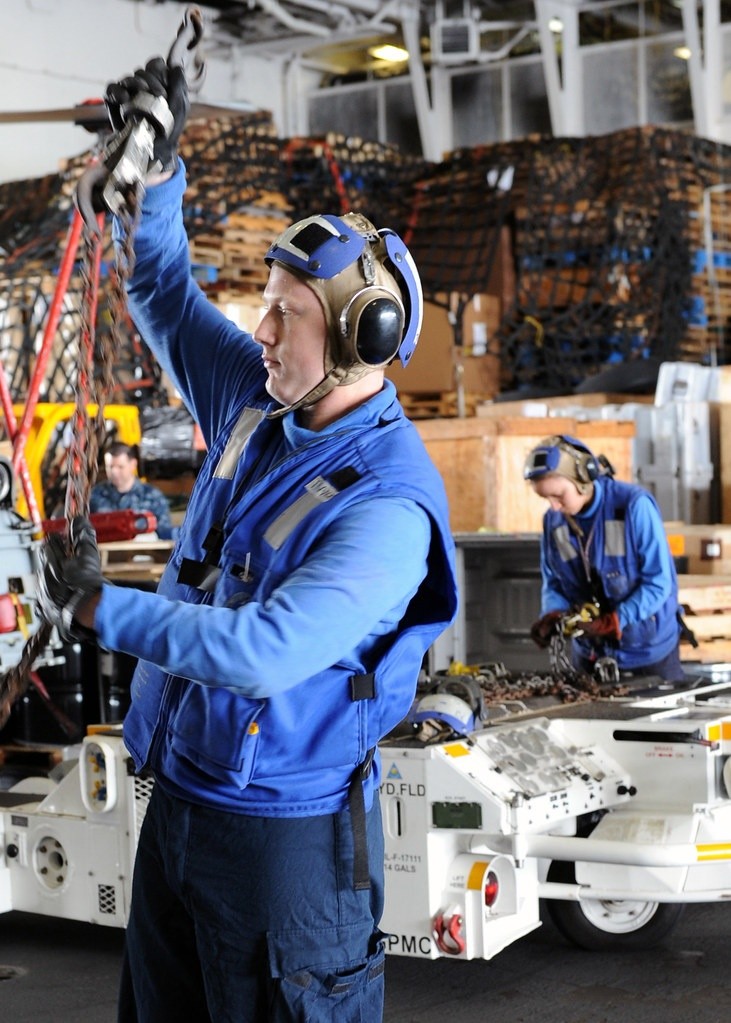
524;434;602;494
409;677;486;739
263;211;423;386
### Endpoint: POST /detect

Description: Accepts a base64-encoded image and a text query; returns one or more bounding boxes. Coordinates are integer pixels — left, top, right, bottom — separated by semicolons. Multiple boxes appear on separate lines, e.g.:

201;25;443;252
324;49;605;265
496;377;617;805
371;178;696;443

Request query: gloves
576;611;622;639
36;515;114;643
532;611;561;648
103;58;189;174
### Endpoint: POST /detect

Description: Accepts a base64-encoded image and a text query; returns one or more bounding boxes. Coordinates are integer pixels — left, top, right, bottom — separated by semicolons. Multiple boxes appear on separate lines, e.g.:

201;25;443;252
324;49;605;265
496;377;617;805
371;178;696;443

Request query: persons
523;434;683;679
37;57;457;1023
90;442;173;538
